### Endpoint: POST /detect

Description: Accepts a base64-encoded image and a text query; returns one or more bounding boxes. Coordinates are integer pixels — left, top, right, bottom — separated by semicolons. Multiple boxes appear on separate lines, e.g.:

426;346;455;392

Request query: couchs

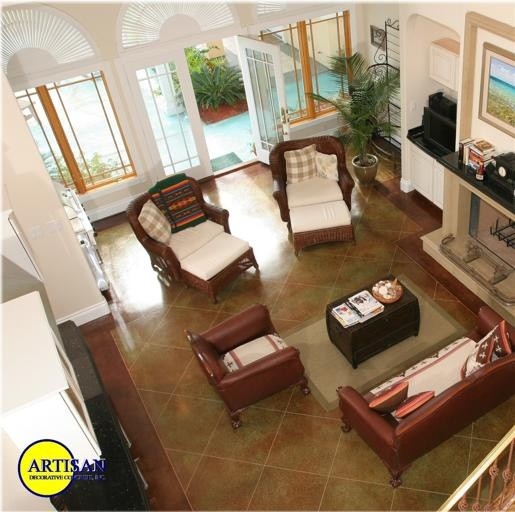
335;306;515;489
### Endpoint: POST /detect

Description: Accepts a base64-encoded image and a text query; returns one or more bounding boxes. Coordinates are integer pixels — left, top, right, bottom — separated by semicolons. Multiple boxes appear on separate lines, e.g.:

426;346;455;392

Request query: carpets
210;152;242;171
279;274;469;413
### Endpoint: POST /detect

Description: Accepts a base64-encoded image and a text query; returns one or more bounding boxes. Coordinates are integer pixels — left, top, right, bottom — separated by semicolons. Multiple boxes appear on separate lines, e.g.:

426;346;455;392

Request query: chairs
124;177;258;304
269;135;355;257
184;304;310;430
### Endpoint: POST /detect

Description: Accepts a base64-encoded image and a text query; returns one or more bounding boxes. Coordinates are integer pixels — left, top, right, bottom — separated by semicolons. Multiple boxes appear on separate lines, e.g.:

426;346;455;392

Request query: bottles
475;161;485;181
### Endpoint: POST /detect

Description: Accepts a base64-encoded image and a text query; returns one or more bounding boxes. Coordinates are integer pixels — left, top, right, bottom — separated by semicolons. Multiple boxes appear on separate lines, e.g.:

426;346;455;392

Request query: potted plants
308;50;400;185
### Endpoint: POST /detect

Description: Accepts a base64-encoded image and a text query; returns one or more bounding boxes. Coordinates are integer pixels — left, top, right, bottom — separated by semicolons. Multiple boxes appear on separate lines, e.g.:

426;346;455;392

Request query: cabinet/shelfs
429;37;460;92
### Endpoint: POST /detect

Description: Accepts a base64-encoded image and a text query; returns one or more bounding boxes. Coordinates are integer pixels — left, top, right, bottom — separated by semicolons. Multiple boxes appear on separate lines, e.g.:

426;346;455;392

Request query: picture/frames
370;25;386;50
478;42;515;139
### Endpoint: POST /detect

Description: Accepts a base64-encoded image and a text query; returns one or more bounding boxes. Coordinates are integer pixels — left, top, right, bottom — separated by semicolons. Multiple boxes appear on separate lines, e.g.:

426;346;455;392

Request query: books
458;137;496;171
331;290;384;328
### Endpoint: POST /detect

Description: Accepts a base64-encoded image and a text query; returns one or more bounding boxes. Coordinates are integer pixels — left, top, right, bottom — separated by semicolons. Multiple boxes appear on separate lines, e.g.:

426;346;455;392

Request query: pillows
474;319;512;358
395;391;434;418
459;335;494;380
283;143;316;184
137;197;172;248
316;150;338;181
369;382;408;414
